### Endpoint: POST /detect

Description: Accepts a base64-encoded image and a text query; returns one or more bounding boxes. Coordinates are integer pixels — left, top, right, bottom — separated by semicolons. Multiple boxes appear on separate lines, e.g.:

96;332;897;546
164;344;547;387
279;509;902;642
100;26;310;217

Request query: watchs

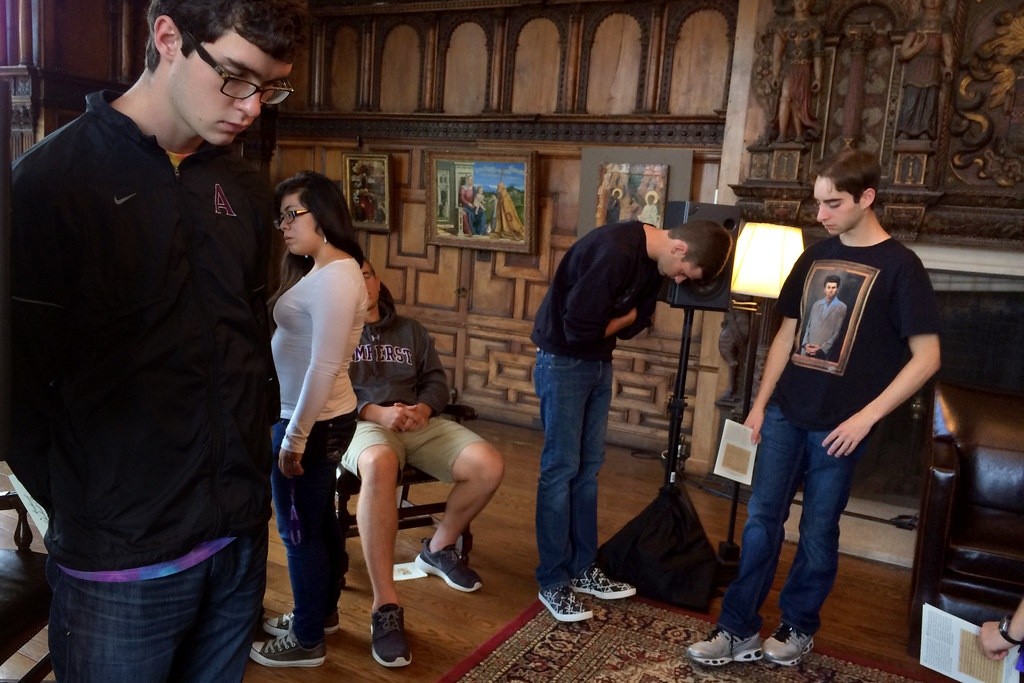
998;617;1022;645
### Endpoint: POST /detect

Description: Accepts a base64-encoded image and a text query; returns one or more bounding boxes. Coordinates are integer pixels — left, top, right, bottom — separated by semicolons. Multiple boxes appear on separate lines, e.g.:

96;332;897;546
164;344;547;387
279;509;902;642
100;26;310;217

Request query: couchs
906;377;1024;660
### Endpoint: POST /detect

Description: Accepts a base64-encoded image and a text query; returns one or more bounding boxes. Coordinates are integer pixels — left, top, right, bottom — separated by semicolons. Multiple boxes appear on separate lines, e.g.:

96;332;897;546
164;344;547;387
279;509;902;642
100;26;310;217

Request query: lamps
717;222;804;588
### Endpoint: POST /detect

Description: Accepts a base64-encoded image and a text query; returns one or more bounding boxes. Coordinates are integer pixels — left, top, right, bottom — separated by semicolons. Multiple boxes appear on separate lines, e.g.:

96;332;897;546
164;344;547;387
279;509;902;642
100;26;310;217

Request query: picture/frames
341;151;394;233
423;147;538;256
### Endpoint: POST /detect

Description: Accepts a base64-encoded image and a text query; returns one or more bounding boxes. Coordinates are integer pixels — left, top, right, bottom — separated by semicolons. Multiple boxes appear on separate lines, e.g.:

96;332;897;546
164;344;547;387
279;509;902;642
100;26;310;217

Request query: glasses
173;17;294;106
277;208;311;226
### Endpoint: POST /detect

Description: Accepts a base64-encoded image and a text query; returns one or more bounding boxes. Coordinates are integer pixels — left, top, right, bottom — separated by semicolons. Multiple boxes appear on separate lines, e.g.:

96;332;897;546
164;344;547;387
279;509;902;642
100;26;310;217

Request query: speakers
655;200;741;312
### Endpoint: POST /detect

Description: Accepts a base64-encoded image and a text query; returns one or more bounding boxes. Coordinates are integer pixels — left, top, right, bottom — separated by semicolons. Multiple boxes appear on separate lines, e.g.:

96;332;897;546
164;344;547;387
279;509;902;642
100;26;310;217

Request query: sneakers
568;565;637;599
538;583;593;622
687;628;763;665
261;608;340;636
248;631;325;667
370;603;411;667
761;623;815;666
415;538;482;592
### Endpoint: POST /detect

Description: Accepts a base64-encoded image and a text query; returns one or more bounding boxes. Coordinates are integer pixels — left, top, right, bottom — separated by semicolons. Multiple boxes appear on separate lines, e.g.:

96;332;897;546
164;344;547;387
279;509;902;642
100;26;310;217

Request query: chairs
333;402;480;589
0;491;54;683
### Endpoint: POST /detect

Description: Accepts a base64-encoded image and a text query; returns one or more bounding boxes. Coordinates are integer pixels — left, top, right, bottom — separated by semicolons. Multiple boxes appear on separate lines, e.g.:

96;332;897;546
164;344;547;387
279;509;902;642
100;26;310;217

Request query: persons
529;219;731;620
977;600;1024;683
686;150;941;668
5;0;304;683
249;170;370;668
338;255;504;667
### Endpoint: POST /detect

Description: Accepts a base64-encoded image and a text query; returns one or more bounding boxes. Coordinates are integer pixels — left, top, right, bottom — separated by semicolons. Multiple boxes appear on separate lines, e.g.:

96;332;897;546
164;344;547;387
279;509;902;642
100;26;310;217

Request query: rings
294;460;301;466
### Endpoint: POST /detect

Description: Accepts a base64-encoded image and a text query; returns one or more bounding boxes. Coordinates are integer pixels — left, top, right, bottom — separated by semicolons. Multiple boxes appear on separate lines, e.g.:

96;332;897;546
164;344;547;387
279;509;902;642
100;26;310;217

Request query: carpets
436;592;945;683
